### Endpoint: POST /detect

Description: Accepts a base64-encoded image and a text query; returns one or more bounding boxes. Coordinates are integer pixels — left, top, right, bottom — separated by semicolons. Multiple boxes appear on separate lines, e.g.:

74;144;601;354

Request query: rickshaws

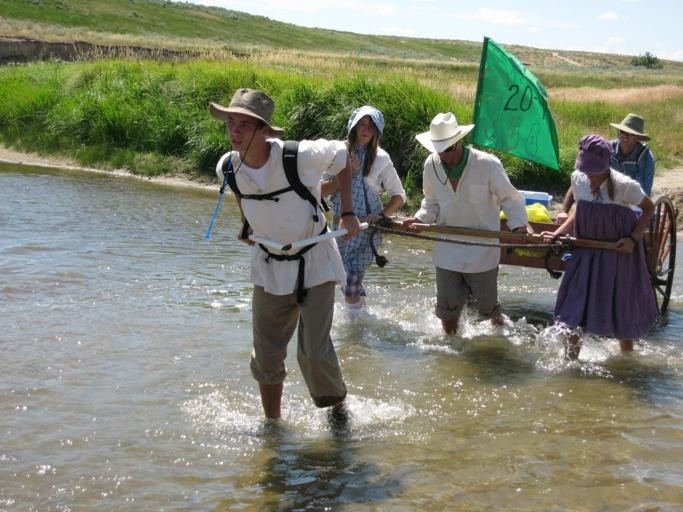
388;195;677;312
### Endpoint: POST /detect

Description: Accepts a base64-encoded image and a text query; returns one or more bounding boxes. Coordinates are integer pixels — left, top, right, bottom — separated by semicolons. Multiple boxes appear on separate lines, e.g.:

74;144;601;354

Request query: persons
319;106;407;303
402;112;529;337
208;88;362;426
539;134;656;363
563;112;655;214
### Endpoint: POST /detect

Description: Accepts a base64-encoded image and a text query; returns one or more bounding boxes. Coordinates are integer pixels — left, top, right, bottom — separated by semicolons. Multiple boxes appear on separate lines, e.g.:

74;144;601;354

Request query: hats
575;134;611;174
415;112;475;154
209;88;286;138
610;113;650;141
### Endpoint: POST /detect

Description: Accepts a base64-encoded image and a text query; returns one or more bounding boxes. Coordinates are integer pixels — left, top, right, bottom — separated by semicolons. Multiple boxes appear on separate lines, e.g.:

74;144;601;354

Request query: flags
469;38;562;174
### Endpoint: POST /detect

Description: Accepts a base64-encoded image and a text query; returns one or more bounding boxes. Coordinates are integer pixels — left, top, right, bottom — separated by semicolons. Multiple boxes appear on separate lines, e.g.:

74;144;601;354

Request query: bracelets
625;234;638;246
340;212;356;218
